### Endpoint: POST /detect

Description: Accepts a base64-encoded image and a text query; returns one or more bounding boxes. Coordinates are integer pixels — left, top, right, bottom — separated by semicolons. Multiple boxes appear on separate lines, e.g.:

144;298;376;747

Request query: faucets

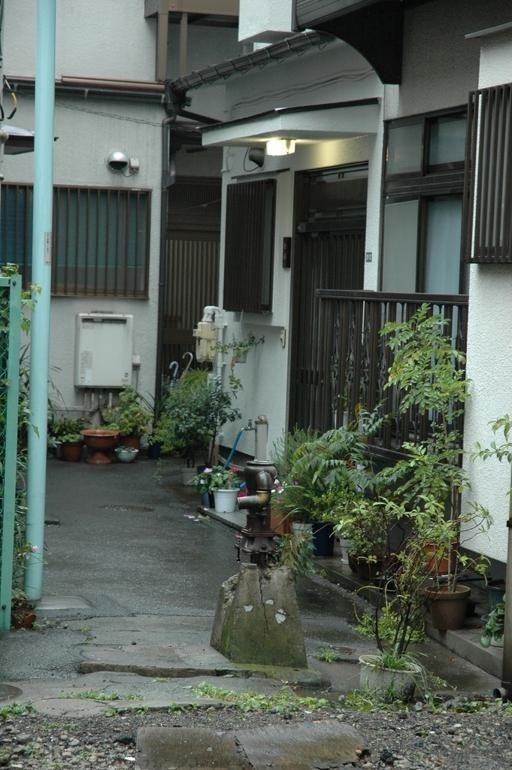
241;417;256;432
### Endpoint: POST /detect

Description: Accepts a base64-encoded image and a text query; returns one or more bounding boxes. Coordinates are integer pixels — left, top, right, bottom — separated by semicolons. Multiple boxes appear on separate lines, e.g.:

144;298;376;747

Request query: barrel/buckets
291;521;314;537
212;486;242;513
339;537;355;564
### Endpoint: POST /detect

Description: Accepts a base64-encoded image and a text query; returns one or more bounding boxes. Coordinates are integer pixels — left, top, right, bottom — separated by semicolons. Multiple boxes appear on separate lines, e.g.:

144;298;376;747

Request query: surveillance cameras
108;152;127;170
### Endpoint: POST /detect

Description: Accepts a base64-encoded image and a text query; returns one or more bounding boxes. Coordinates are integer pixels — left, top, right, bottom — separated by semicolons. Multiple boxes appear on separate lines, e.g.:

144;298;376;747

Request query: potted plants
48;385;153;465
380;303;487;630
189;464;223;509
349;507;492;704
194;405;456;583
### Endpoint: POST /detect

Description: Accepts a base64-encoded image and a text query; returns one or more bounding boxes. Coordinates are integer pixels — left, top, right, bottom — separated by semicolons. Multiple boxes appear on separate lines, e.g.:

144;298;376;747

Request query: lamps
265;140;296;156
